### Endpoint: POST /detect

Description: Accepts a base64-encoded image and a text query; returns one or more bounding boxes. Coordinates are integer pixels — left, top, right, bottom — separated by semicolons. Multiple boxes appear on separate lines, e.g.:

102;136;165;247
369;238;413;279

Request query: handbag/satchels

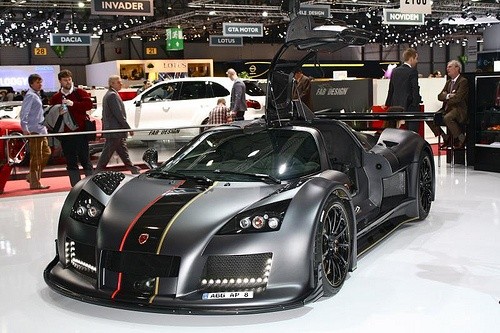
85;119;96;141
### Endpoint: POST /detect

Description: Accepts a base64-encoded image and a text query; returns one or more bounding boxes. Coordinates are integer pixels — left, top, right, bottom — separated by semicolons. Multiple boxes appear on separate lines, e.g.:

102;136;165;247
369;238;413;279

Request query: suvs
90;76;266;141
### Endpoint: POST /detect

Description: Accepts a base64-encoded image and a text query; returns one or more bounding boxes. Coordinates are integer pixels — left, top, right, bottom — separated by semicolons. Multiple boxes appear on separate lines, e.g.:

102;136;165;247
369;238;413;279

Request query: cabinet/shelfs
470;73;500;173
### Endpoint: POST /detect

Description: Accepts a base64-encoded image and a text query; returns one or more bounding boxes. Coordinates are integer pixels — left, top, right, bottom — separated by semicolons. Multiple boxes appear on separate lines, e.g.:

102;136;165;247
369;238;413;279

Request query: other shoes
439;141;448;150
453;141;464;149
30;186;50;189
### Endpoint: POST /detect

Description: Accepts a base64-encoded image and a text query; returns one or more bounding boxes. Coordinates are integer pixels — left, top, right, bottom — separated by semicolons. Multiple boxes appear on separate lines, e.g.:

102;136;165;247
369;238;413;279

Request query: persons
96;76;140;176
50;70;94;185
208;98;232;127
385;106;405;129
426;60;469;149
385;48;421;132
292;69;311;108
0;90;28;102
137;81;150;95
227;68;247;121
433;70;442;78
20;74;52;190
39;89;48;105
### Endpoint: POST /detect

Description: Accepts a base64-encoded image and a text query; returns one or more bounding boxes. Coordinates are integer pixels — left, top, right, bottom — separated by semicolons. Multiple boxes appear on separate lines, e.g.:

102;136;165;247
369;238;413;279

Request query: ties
445;81;454;111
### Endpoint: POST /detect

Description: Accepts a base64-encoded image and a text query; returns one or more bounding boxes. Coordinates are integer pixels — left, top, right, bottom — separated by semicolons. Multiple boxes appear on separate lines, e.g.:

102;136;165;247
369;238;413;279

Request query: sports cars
43;0;443;315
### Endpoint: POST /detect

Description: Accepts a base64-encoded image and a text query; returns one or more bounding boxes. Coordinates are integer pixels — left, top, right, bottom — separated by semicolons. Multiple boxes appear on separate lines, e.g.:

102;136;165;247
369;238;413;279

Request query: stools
437;124;469;170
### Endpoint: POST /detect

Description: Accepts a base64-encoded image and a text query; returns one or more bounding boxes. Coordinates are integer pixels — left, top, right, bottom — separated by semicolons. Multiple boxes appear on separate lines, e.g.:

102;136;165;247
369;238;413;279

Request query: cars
0;101;106;168
